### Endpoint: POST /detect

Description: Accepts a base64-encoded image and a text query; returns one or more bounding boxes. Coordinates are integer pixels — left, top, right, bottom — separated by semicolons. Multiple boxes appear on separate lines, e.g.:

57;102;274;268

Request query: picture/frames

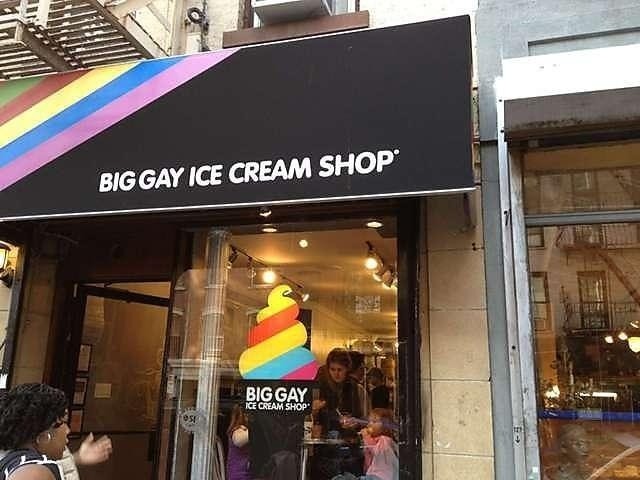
73;376;89;406
76;344;92;373
68;408;84;438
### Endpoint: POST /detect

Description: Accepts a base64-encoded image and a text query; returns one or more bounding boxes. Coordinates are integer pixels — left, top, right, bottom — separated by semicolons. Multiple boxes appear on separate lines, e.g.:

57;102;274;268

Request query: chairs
212;434;227;480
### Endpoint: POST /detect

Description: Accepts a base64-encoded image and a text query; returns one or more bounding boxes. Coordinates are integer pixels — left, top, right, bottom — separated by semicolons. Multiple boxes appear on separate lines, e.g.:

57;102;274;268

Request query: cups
311;425;321;441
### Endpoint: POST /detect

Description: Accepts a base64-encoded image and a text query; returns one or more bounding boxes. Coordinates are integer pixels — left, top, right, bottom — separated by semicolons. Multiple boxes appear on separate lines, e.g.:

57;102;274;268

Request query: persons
547;424;592;479
0;384;113;480
226;348;399;480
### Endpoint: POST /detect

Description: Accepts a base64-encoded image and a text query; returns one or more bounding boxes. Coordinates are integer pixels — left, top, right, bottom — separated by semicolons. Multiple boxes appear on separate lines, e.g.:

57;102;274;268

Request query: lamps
366;241;399;290
259;205;272;218
225;245;310;302
0;241;15;289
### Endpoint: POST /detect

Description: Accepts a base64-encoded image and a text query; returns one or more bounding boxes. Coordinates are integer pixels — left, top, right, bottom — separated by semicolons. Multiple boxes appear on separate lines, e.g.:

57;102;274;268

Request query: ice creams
238;284;320;381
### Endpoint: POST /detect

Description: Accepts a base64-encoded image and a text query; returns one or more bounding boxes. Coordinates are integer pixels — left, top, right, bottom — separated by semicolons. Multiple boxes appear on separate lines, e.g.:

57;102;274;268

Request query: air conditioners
251;0;336;25
